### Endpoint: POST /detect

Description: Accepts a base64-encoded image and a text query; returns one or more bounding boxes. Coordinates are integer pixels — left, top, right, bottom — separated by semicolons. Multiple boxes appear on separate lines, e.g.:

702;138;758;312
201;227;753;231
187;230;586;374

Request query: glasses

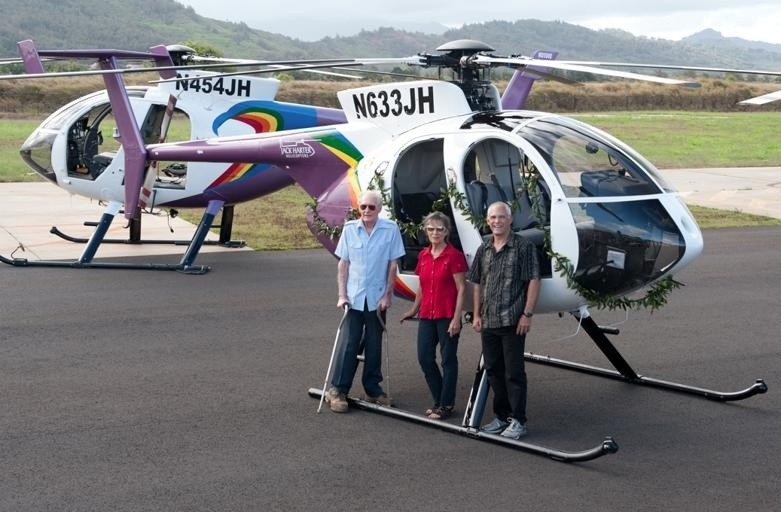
427;227;445;232
359;204;377;211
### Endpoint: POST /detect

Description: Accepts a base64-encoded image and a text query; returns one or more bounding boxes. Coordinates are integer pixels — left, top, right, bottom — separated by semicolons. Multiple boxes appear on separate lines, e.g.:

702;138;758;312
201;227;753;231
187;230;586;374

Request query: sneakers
500;417;529;440
359;388;393;408
479;416;510;435
324;383;349;413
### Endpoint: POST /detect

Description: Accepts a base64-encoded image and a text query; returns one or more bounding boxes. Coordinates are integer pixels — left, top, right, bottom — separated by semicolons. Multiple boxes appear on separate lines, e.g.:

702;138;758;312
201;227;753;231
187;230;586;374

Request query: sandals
424;404;456;420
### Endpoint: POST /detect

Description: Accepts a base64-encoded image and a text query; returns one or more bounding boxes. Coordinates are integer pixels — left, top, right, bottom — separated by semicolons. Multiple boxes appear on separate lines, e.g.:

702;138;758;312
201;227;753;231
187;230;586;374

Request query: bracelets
523;311;533;318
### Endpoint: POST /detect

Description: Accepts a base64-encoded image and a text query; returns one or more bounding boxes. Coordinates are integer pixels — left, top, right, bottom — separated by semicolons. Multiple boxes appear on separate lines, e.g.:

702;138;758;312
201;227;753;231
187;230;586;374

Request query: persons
467;201;542;439
324;189;406;414
399;211;469;421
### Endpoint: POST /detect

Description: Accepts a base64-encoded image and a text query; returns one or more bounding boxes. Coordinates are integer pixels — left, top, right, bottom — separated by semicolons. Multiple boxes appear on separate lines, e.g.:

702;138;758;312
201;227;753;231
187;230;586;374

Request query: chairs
467;179;546;263
503;174;550;230
90;150;116;165
400;191;459;251
